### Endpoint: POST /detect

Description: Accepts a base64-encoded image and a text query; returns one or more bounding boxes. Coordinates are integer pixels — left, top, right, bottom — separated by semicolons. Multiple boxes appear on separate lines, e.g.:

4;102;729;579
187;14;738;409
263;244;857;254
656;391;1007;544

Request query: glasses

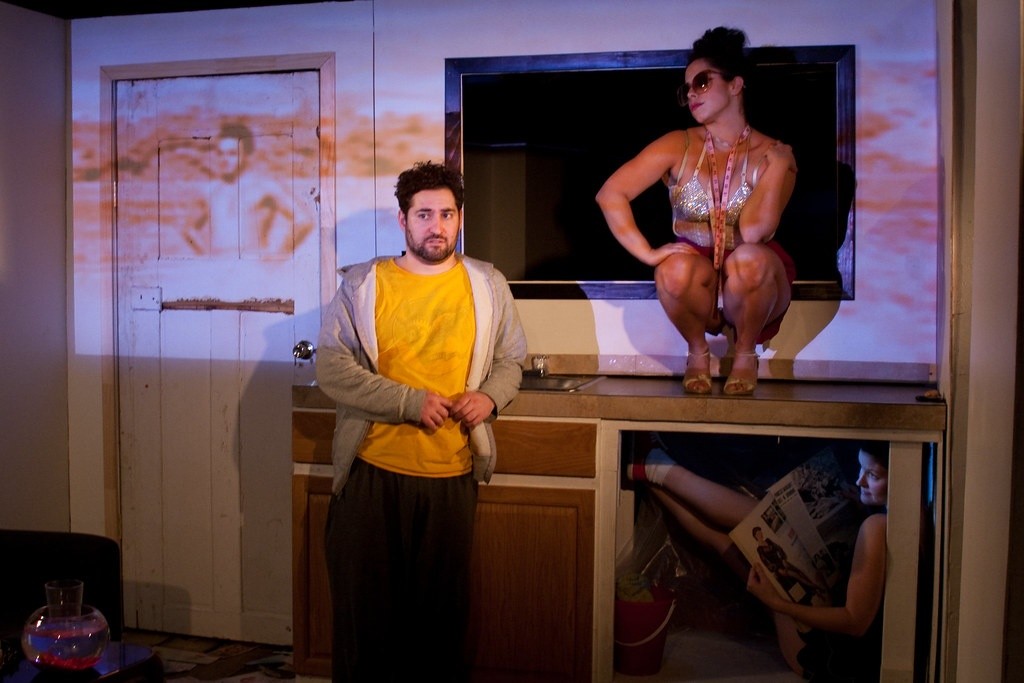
677;70;722;107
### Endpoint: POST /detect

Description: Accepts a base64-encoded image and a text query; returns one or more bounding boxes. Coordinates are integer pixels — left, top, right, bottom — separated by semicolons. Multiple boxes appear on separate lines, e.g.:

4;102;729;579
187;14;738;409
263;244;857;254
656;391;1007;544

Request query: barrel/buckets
613;580;677;677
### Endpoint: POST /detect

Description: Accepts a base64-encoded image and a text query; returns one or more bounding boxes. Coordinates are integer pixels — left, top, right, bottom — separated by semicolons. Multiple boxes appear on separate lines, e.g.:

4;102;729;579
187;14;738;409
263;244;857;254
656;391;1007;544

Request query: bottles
21;578;110;669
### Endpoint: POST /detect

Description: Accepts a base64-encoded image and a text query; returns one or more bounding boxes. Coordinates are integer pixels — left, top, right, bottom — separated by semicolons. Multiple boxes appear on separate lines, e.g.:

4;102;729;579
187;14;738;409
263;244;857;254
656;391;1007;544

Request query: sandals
682;347;711;394
725;351;759;397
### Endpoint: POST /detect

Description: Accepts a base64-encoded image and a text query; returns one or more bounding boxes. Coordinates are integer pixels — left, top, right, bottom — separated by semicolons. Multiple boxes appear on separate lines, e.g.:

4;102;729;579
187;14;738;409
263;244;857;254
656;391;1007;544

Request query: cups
531;354;549;377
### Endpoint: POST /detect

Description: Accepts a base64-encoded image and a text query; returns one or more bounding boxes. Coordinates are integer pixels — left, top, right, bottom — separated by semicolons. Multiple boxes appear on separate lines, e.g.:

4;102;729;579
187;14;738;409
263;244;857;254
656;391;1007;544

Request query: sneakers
627;430;667;486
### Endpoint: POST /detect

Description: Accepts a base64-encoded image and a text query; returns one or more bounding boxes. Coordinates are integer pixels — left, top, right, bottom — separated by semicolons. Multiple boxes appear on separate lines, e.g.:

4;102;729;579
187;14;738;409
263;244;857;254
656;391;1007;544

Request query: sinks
519;376;609;392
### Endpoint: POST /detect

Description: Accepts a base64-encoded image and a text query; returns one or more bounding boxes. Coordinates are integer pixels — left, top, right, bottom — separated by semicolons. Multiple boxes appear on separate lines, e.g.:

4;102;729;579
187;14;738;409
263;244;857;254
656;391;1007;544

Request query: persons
752;525;825;595
316;160;527;683
623;429;886;683
594;25;798;398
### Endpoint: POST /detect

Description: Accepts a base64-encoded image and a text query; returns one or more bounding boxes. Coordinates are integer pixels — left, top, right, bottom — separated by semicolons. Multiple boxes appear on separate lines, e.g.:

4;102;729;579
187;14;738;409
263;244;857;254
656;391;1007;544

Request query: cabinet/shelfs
289;373;948;682
